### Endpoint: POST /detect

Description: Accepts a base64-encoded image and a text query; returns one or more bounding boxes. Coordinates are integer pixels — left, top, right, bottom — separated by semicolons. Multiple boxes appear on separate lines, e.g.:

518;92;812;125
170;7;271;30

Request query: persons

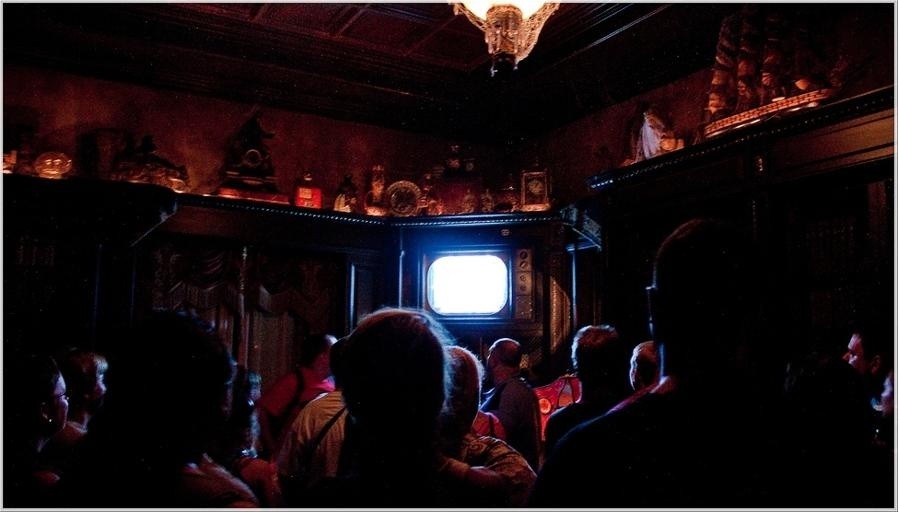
3;218;894;507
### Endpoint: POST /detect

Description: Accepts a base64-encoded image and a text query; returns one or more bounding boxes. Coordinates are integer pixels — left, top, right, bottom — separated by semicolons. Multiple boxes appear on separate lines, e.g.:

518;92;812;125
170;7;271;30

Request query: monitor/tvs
416;242;537;325
